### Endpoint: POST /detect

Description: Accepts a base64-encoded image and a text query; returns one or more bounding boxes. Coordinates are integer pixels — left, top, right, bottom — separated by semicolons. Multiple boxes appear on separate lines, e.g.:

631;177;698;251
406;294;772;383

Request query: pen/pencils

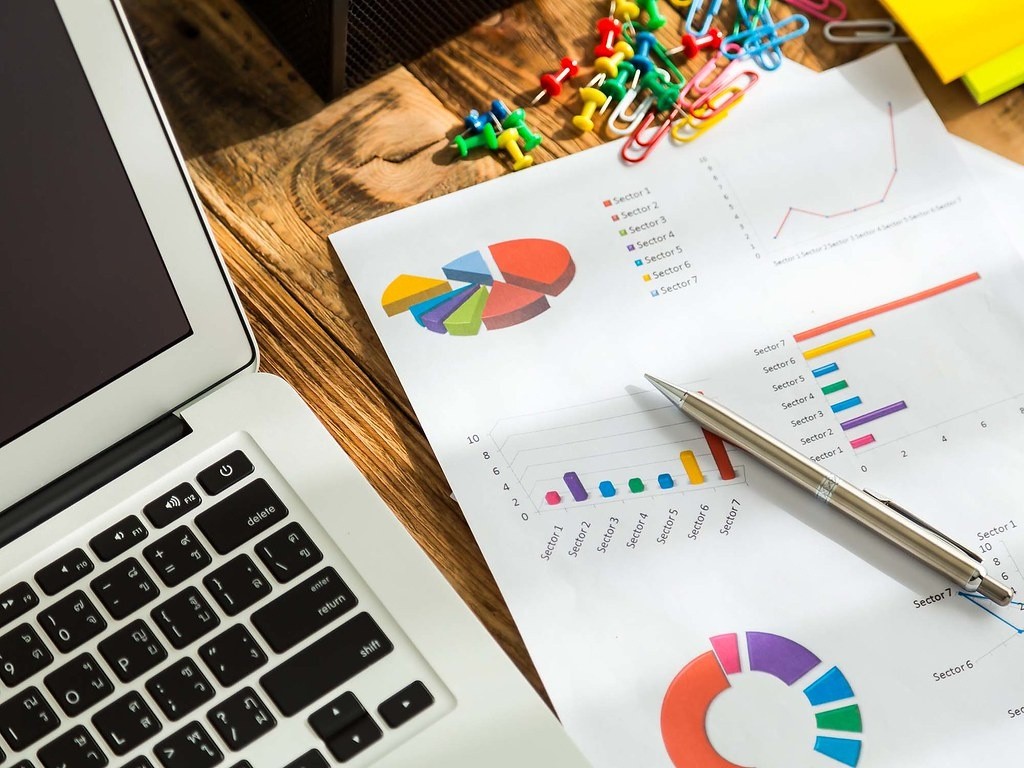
642;367;1017;609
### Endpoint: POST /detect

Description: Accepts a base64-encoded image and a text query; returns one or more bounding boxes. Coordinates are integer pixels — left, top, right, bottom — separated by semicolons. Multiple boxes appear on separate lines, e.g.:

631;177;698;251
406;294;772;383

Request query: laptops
0;0;590;768
